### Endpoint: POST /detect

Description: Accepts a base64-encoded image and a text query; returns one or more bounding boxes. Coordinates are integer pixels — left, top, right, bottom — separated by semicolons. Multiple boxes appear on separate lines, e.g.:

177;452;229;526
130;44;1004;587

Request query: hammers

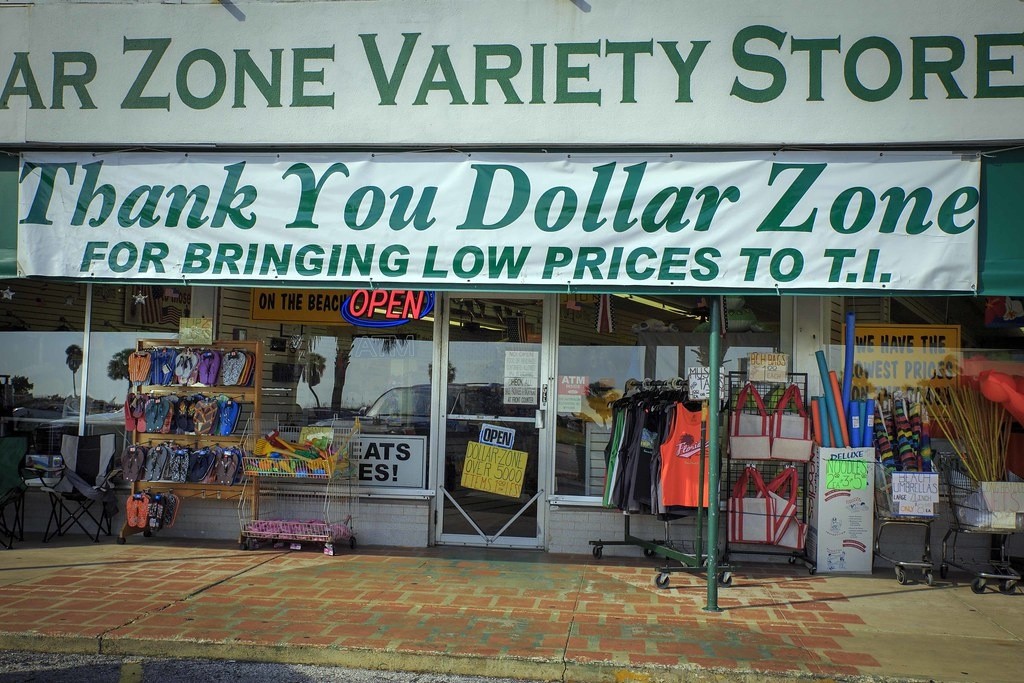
265;429;296;452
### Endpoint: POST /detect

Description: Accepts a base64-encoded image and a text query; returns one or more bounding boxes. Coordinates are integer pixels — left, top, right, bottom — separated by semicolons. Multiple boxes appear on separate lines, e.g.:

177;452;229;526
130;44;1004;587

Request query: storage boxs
808;443;876;576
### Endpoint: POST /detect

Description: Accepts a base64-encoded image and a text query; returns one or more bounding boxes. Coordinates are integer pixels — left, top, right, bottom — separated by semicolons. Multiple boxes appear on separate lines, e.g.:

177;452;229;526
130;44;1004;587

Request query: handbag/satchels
725;382;807;549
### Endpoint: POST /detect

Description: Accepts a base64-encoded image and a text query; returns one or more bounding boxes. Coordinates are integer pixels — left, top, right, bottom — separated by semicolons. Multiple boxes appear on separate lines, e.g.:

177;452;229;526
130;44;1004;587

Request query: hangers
607;378;711;414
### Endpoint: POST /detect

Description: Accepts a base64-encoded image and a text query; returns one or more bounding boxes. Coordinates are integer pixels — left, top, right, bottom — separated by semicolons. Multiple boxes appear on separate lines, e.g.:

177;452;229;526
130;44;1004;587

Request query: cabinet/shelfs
117;339;262;549
238;414;361;556
726;370;818;572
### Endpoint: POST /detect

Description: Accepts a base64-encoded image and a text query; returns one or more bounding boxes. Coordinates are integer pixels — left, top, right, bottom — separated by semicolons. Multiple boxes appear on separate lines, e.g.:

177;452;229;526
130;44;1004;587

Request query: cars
12;404;125;483
309;383;586;506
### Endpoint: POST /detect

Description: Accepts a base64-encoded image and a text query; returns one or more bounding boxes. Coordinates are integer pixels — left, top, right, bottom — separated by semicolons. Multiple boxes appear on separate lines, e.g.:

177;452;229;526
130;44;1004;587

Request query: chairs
41;434;119;543
0;435;27;549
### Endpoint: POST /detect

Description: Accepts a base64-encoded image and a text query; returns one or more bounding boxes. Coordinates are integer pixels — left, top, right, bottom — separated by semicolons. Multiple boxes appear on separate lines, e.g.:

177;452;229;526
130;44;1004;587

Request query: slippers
249;517;346;538
120;344;256;532
